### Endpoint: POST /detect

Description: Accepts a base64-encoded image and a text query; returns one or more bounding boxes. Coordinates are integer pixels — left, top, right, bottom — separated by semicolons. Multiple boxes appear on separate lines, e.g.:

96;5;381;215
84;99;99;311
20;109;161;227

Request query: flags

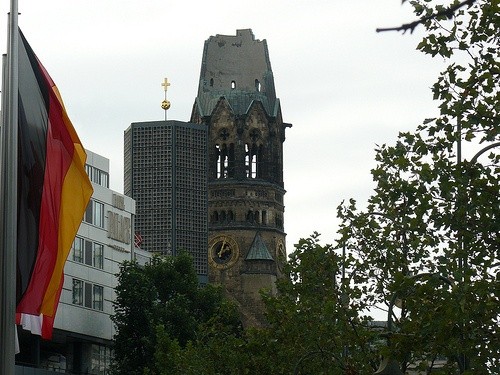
15;27;95;355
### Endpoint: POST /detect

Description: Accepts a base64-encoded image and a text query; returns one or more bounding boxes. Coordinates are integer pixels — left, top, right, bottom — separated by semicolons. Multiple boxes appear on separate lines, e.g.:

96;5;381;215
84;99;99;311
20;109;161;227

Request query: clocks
276;237;287;275
207;232;240;270
